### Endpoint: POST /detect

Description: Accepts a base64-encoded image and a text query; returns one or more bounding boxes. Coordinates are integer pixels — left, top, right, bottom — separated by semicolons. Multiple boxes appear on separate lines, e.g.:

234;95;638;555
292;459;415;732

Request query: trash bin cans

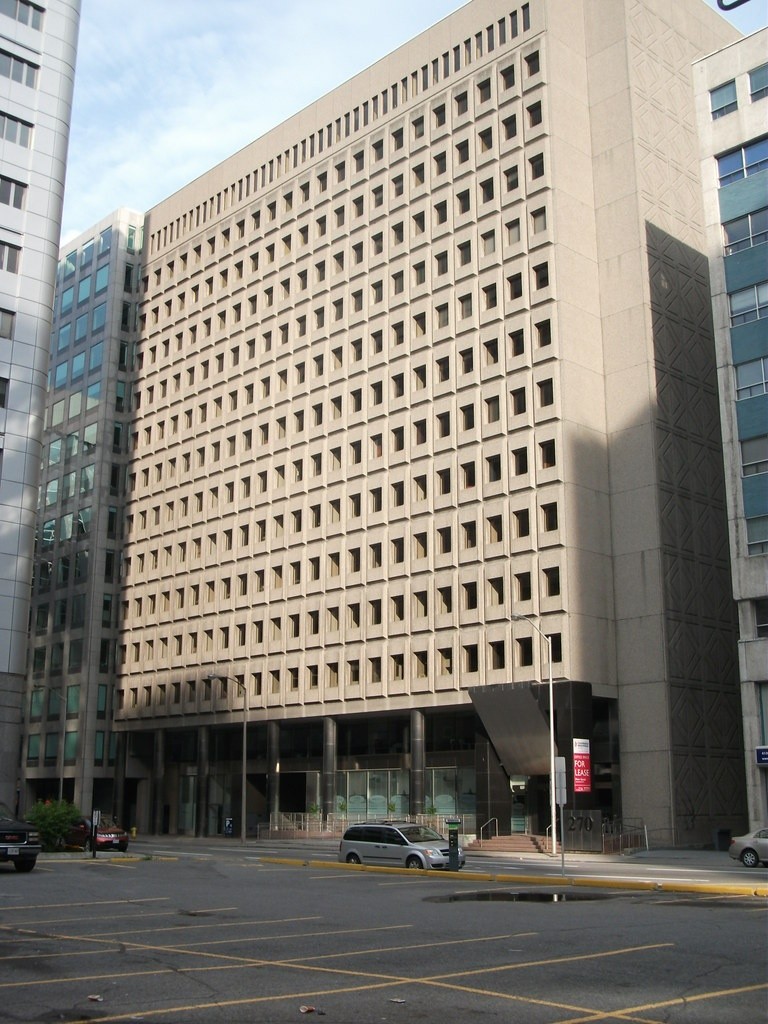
711;828;731;851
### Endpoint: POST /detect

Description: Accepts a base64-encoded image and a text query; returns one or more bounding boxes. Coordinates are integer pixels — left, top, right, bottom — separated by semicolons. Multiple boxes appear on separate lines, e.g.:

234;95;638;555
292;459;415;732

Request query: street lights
207;672;248;843
510;612;557;857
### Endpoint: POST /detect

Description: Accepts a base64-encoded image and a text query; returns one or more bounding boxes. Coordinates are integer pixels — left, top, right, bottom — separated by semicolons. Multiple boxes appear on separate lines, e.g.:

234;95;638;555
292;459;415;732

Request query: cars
66;815;129;852
337;819;465;872
727;827;768;867
0;811;42;873
